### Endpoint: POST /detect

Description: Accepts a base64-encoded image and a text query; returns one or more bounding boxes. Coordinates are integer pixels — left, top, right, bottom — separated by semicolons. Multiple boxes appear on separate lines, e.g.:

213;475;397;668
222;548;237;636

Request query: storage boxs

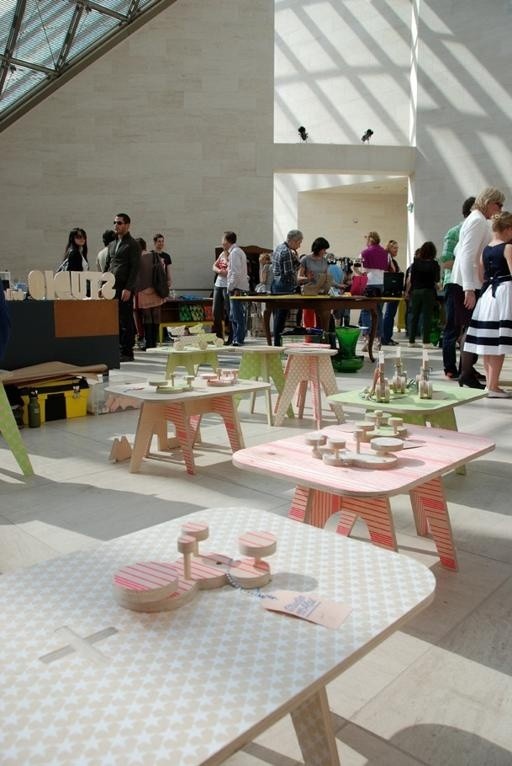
17;376;91;425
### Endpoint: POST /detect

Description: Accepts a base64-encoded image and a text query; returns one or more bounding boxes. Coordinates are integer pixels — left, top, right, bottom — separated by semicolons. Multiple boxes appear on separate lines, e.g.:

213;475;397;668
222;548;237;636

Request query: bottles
28;390;41;427
375;351;390;402
418;349;433;398
393;347;405;393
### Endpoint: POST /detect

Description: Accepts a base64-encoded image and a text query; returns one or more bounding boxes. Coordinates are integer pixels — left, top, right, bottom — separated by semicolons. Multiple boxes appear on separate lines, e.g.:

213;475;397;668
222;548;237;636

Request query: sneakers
408;342;432;348
120;354;134;362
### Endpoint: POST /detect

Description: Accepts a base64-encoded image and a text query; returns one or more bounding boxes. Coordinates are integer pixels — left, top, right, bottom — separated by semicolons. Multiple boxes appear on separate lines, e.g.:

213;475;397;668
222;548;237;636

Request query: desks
1;506;437;766
229;290;389;364
104;375;271;476
325;376;489;476
146;343;239;385
230;421;496;573
158;299;213;345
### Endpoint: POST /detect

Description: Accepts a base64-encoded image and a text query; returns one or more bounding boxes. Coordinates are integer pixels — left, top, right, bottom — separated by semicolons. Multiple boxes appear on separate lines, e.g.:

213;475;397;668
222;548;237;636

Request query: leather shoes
447;370;486;389
362;335;399;351
488;389;511;398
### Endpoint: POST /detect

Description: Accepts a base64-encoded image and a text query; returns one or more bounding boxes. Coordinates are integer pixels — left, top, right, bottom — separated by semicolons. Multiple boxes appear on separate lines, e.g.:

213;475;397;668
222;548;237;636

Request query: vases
331;325;364;372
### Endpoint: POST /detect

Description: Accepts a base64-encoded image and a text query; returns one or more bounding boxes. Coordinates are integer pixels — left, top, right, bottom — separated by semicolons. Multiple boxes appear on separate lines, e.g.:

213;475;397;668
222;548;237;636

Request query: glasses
114;221;125;225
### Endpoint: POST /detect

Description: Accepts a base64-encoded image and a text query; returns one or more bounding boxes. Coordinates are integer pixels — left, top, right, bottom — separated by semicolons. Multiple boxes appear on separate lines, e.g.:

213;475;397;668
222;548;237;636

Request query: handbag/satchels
56;258;71;271
152;263;169;298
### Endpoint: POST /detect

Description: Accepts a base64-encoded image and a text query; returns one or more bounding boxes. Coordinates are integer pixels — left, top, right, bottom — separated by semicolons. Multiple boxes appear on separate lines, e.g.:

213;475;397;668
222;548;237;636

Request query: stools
273;343;348;431
233;345;295;419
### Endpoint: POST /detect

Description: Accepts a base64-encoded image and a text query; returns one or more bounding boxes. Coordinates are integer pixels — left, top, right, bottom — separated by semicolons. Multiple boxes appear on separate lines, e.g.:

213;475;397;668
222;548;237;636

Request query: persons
441;197;477;378
95;213;173;360
450;184;506;389
208;229;444;351
462;210;512;398
56;227;89;272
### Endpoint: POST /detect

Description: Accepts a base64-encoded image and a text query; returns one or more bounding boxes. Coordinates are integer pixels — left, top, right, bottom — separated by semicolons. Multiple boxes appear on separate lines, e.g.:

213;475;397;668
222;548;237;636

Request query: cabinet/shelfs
215;246;273;294
